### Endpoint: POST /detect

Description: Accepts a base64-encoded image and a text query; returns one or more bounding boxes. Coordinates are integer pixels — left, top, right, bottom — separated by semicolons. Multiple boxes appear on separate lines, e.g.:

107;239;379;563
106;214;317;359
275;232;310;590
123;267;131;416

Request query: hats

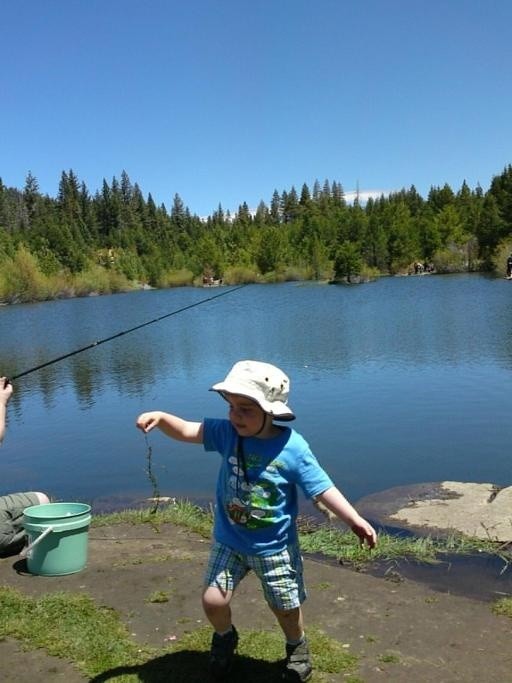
209;360;296;421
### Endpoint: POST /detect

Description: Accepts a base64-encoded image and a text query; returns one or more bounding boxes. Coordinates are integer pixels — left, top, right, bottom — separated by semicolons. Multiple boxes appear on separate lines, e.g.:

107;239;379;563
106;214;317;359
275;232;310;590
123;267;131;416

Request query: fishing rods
5;269;288;382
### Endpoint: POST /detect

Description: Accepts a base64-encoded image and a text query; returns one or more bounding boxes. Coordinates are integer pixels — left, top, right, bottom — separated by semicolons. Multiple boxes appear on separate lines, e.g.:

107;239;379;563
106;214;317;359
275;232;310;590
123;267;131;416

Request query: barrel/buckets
20;503;91;576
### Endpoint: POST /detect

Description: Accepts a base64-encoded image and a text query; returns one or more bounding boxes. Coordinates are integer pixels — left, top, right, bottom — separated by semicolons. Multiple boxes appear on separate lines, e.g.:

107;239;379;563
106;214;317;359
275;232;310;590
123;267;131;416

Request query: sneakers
206;625;239;678
282;636;314;682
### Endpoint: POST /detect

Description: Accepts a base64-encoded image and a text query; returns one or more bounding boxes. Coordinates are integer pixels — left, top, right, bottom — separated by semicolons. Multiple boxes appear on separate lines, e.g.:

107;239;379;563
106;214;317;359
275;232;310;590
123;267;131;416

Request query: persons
136;359;377;683
0;377;50;559
